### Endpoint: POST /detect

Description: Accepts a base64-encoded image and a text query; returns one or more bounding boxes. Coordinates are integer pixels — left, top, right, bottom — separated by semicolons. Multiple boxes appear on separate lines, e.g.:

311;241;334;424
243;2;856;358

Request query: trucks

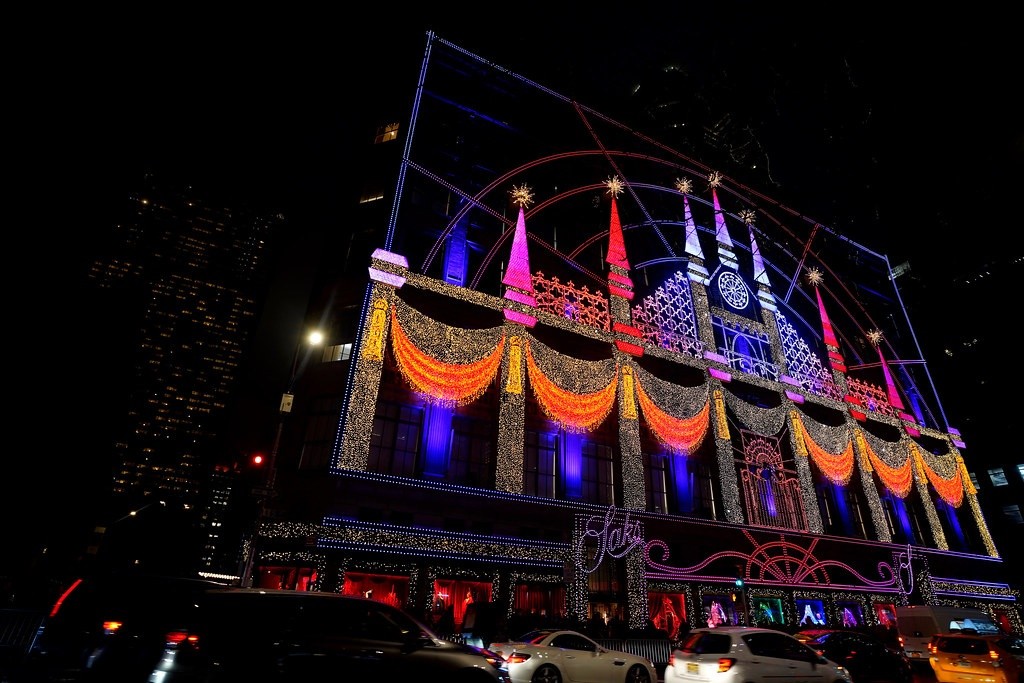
890;605;1007;661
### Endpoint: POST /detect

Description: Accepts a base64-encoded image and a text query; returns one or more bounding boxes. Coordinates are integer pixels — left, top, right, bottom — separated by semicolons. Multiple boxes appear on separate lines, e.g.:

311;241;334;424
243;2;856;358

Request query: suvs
74;588;515;683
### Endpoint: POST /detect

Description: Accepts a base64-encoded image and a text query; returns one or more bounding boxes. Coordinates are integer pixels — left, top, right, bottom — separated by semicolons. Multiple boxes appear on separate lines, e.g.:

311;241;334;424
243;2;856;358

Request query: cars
926;633;1024;683
663;624;854;683
487;629;659;683
792;628;918;683
20;564;243;683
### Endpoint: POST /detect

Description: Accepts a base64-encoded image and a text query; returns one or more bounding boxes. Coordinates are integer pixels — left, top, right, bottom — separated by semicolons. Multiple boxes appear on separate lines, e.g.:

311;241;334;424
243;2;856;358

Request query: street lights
238;324;334;590
